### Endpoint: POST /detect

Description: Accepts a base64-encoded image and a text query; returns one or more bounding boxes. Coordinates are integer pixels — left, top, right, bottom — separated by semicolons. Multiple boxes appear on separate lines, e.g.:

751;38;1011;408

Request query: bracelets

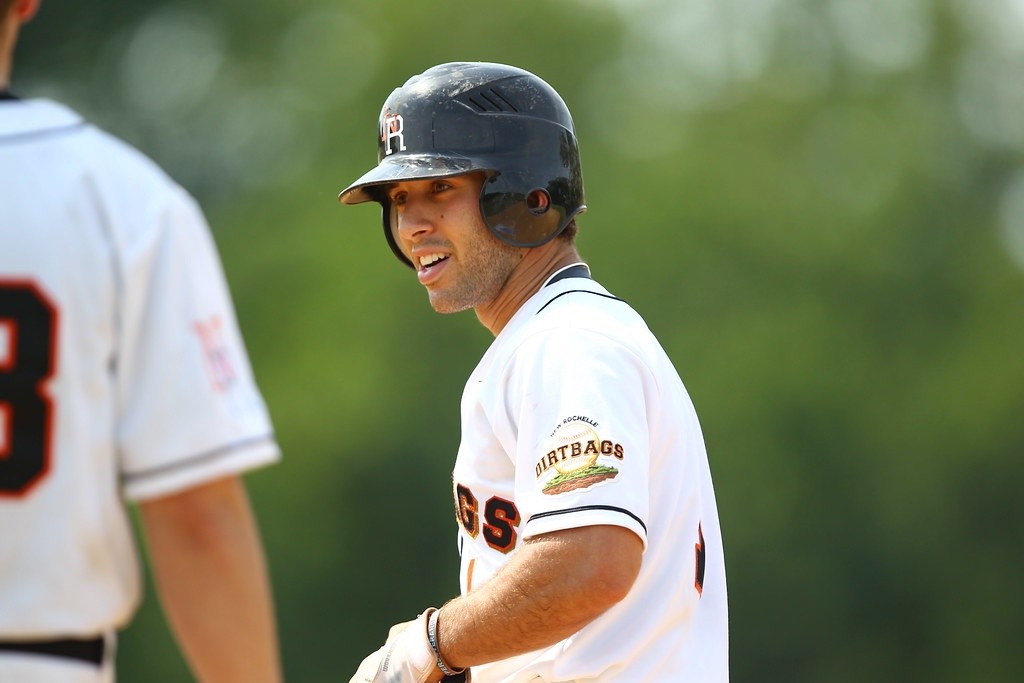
428;610;466;676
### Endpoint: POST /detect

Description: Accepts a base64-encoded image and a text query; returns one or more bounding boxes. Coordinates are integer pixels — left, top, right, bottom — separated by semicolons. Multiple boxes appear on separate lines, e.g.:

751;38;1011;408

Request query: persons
337;61;731;683
0;0;285;683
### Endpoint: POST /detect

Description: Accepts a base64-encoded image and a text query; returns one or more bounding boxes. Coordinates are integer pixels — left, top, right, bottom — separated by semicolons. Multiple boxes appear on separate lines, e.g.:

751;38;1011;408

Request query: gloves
350;607;471;683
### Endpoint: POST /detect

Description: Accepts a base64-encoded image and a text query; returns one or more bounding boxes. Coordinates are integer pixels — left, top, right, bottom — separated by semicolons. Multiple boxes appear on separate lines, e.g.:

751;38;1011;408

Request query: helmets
338;62;586;269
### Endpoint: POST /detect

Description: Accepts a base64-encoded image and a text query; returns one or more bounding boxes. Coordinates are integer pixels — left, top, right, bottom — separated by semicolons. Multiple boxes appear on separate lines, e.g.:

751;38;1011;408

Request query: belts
0;637;106;664
